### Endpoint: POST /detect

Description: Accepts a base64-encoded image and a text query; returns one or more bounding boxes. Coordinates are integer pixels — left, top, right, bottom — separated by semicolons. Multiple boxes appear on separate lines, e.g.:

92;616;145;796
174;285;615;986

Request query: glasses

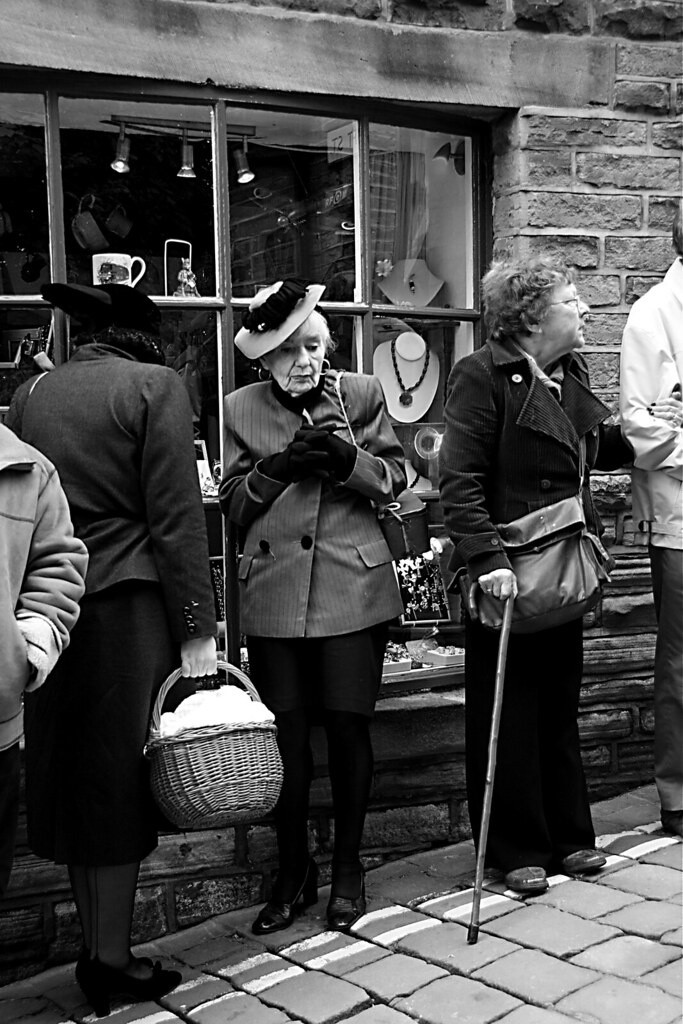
542;295;580;313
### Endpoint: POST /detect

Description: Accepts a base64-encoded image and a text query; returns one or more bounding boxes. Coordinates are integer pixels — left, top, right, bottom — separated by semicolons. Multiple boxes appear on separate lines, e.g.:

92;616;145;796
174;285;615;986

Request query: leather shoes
503;865;548;891
561;848;607;872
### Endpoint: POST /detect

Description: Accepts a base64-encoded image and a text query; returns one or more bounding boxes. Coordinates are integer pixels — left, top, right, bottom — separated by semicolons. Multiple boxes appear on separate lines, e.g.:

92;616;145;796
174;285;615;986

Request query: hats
235;280;327;360
39;283;162;335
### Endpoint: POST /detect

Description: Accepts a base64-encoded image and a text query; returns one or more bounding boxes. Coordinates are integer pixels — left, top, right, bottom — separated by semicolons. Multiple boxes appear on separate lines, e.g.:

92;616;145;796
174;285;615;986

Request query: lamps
176;130;196;179
234;136;256;184
431;141;465;173
109;123;130;173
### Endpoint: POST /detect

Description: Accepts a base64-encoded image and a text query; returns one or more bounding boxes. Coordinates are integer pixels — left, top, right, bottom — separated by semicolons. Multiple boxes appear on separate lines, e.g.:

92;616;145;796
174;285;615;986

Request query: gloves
263;422;357;484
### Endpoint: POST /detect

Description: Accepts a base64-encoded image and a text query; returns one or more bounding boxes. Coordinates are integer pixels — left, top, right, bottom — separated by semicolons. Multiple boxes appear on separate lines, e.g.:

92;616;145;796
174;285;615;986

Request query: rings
673;414;676;419
487;589;493;592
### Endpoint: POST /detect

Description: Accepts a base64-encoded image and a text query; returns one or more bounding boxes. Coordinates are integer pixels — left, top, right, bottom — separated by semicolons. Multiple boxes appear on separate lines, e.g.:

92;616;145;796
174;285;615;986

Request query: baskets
144;660;284;830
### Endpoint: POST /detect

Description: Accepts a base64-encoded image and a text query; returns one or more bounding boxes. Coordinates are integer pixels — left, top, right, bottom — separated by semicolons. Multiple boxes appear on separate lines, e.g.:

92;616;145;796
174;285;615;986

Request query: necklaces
409;473;421;489
391;338;430;406
389;270;428;294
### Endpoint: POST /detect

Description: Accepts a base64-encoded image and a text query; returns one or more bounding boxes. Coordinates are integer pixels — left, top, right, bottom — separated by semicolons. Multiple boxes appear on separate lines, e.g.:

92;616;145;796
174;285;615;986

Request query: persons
177;259;201;297
620;208;683;838
0;422;88;904
5;283;216;1018
437;249;683;893
219;282;407;935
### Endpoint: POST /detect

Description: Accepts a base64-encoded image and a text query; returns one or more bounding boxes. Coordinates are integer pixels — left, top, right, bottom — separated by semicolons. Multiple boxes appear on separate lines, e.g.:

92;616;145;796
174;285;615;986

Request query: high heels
251;856;320;935
75;954;183;1018
325;862;368;931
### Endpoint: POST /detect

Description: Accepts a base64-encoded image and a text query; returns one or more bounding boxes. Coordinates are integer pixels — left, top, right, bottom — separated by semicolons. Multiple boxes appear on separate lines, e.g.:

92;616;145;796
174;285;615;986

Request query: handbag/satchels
376;501;432;560
460;493;613;637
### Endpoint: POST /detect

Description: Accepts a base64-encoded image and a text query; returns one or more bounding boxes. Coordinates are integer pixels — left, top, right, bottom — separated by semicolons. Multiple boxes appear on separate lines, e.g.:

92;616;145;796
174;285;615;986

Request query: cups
105;204;133;238
71;196;107;253
93;253;147;286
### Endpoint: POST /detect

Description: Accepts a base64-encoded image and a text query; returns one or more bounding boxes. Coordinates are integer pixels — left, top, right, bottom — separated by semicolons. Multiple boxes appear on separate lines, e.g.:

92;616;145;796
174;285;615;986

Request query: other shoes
660;809;683;838
